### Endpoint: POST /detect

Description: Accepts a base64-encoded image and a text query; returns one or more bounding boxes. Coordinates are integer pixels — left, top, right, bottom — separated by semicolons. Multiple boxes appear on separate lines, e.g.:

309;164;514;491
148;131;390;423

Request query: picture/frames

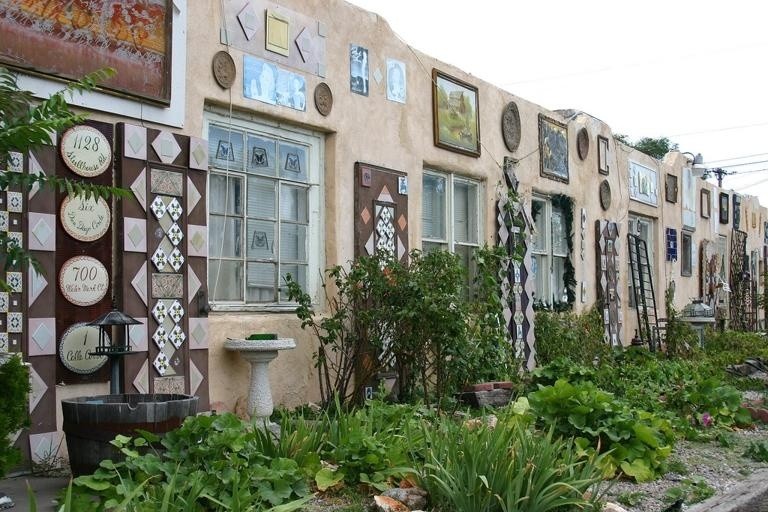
431;67;482;158
700;188;712;219
719;191;729;225
536;112;571;184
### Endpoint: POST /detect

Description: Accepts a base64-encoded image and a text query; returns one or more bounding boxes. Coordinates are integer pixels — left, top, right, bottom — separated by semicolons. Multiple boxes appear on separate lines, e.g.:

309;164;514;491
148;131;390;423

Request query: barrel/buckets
61;394;197;481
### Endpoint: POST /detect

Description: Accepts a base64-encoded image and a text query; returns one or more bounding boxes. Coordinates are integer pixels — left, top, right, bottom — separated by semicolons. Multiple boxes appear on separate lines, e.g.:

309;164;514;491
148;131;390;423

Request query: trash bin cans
682;299;715;350
